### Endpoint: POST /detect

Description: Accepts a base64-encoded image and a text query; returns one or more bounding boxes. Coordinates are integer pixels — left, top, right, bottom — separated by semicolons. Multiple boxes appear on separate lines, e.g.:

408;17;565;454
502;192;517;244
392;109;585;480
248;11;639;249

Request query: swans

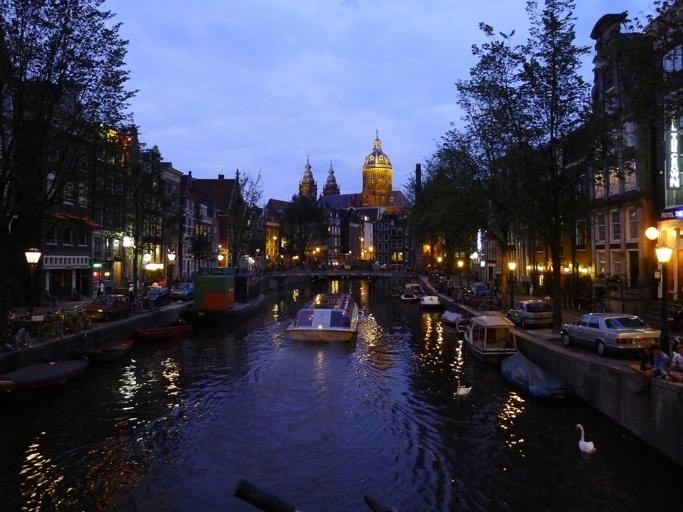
576;423;596;453
457;380;471;396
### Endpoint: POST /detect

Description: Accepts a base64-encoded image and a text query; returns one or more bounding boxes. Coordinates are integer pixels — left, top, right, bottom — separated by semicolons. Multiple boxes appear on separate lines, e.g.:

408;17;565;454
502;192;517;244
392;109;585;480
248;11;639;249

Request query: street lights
437;257;443;276
507;262;517;310
457;260;464;286
217;254;224;267
167;254;176;298
25;247;41;312
655;242;673;357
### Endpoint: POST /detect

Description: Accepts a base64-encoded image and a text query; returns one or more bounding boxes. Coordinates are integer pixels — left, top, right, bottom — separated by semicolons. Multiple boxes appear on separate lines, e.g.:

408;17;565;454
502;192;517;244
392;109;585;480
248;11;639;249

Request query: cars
135;282;194;309
81;294;132;322
560;312;662;357
507;299;554;329
433;275;501;310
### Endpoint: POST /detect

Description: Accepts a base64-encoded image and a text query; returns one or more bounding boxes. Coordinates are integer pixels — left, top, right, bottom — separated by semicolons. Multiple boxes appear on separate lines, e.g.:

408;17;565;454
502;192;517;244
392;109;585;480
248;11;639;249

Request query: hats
672;335;683;353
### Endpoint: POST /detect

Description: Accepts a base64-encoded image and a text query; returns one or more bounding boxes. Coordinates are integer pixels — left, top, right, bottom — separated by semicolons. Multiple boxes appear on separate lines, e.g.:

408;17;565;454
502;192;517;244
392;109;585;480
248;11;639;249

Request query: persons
666;336;682;384
630;343;654;375
632;342;671;396
91;278;106;306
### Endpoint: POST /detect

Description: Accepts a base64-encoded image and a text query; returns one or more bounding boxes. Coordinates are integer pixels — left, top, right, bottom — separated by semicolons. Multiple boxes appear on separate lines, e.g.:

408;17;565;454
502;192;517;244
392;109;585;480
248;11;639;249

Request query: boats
0;356;95;392
405;283;443;310
83;338;135;361
440;310;465;327
463;314;520;363
499;353;569;399
286;292;358;341
135;323;193;339
197;291;267;317
